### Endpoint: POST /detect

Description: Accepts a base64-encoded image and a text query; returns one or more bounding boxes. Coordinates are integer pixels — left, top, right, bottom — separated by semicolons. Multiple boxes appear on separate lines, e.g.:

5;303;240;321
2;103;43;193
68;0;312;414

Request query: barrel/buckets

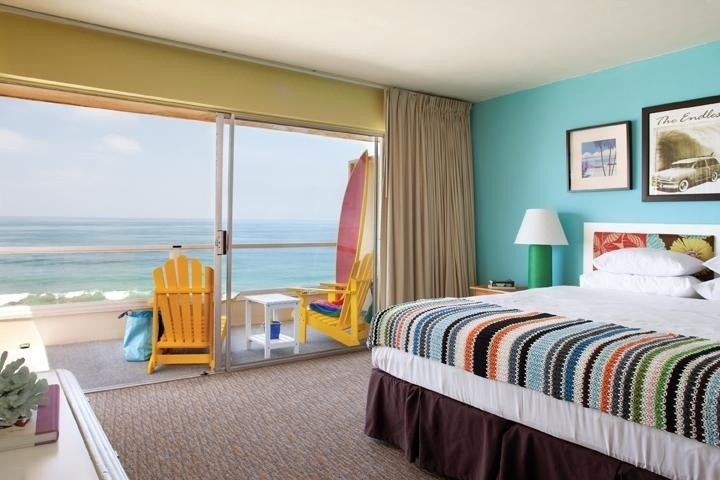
263;319;281;340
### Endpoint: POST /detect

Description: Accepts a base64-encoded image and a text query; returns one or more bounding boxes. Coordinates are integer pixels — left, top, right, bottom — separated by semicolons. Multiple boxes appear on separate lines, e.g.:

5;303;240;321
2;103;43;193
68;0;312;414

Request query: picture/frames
565;121;633;193
642;95;720;202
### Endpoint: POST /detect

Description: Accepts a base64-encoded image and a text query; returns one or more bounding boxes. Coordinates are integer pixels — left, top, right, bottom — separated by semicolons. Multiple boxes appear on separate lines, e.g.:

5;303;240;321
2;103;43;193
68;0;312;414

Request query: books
0;383;60;453
487;279;516;290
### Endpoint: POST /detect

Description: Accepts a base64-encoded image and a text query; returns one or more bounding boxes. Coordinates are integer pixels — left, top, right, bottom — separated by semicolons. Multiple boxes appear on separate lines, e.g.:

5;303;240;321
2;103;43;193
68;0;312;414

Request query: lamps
513;209;569;289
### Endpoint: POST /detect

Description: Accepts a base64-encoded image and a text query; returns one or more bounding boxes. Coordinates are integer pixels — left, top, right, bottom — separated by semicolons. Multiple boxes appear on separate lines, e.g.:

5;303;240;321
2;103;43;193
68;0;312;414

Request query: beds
365;222;720;480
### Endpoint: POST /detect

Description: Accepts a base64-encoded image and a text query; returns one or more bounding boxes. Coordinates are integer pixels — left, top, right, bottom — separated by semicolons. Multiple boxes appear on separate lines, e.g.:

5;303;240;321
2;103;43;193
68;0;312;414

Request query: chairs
147;255;227;371
286;252;372;347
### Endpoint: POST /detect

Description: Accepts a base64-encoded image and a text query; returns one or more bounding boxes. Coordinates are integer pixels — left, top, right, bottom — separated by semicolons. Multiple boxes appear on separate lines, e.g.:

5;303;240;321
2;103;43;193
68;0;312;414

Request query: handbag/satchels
118;309;154;362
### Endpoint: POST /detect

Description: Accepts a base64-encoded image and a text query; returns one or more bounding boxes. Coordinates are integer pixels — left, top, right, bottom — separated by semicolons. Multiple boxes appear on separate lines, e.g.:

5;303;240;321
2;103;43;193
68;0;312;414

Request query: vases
264;320;282;339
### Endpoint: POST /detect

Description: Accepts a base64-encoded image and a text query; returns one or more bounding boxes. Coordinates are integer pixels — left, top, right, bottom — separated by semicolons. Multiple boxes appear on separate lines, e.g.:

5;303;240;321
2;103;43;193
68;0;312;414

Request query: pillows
693;277;720;301
701;255;720;276
591;248;706;276
580;271;703;299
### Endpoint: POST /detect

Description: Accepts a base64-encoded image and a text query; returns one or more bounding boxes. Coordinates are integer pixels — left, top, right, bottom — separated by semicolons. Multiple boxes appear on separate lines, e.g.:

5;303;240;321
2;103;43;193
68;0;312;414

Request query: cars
654;154;720;192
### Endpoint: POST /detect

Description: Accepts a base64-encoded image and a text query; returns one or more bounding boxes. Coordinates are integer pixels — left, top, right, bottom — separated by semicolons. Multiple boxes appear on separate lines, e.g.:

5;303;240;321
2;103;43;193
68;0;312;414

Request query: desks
244;294;301;359
0;369;129;479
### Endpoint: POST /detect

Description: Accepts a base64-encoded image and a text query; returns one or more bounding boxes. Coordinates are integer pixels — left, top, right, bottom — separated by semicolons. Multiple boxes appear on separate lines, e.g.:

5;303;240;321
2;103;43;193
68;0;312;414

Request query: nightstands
470;284;526;295
